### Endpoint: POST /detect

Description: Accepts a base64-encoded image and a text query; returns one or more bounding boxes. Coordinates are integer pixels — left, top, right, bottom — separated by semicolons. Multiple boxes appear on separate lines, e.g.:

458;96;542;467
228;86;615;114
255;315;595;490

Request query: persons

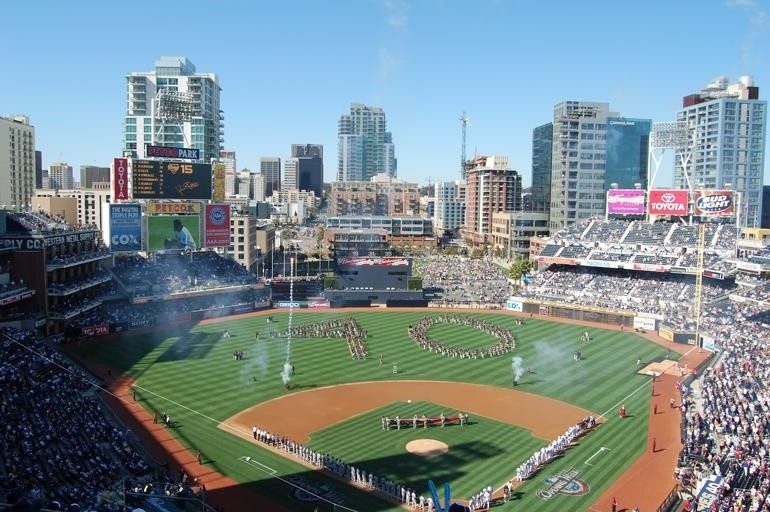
384;415;389;429
380;415;384;431
394;414;400;430
617;404;626;421
244;423;438;512
630;317;770;512
439;413;447;428
2;202;270;343
232;350;246;361
2;337;206;510
573;329;592;363
412;413;419;430
265;314;371;362
458;411;466;427
273;250;516;306
515;214;770;318
404;309;521;362
465;412;599;512
421;414;428;427
610;496;618;512
